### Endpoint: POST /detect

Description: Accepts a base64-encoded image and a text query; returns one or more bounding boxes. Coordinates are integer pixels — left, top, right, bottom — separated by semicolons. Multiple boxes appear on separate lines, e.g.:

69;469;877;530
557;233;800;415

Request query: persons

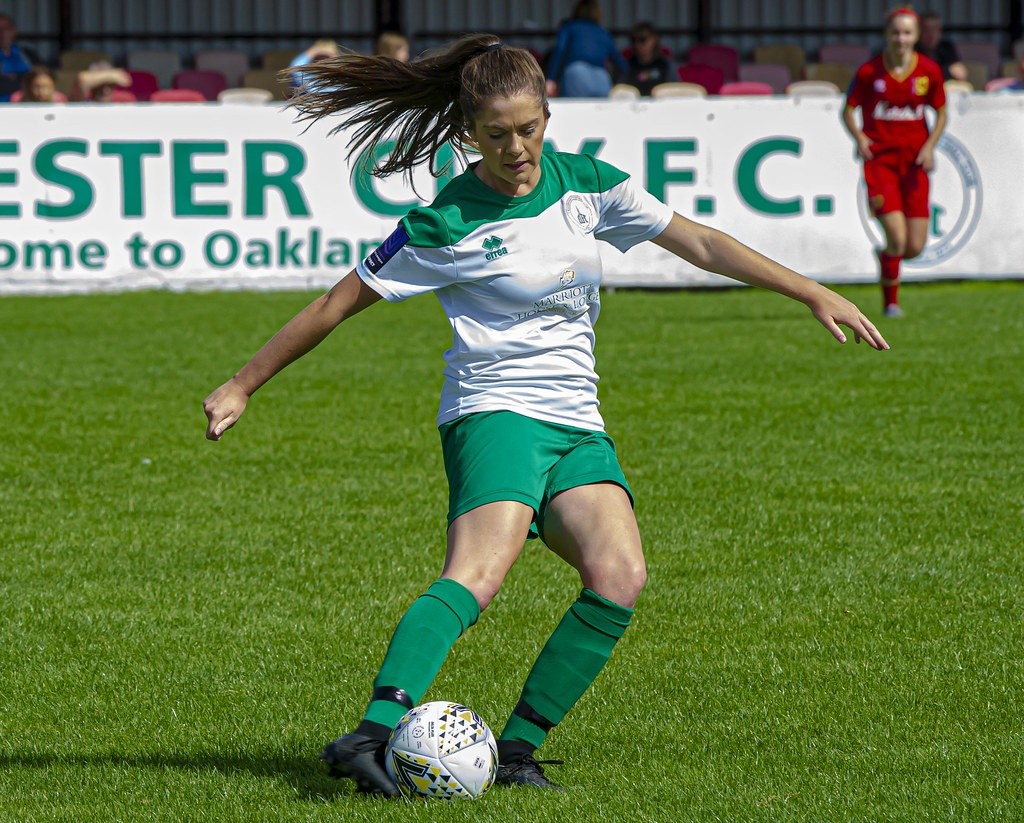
0;0;1024;104
203;34;891;799
843;7;946;318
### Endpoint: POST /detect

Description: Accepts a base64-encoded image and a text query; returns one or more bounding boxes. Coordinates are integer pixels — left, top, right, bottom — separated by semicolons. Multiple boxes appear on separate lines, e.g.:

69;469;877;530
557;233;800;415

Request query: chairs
32;39;1024;102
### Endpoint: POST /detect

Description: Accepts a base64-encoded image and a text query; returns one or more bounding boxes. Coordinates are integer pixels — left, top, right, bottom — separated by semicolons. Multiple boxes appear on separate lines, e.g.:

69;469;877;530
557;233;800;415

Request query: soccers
384;701;499;801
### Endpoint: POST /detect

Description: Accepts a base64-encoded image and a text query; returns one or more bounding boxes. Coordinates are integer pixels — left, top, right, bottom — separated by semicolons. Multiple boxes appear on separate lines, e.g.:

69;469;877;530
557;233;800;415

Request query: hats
633;20;658;41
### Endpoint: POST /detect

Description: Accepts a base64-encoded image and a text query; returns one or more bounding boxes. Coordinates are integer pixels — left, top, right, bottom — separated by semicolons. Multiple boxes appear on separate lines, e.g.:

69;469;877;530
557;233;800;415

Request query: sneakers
495;746;565;790
327;734;405;801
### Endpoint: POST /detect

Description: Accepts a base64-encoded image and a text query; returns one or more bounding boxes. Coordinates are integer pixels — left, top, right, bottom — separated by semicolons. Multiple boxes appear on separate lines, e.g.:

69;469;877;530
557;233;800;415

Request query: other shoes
881;302;907;320
